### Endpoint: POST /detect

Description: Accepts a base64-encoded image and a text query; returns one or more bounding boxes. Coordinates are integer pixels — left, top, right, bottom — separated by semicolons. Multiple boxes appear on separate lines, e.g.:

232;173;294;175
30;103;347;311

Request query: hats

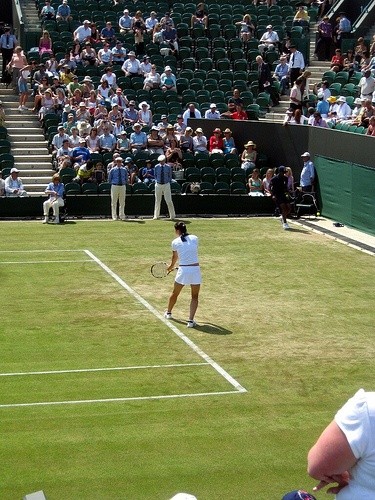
68;113;74;118
2;27;10;32
244;141;256;149
209;103;216;108
83;76;93;82
10;167;20;174
164;66;172;71
326;95;337;102
194;128;204;134
79;102;86;106
77;122;90;131
223;128;232;134
353;98;362;103
185;127;193;133
121;131;127;135
337;95;346;102
125;157;132;163
115;157;123;161
266;25;272;29
149;126;160;131
128;100;135;105
127;51;135;56
301;152;310;157
213;128;221;132
138;101;150;109
116;88;122;93
132;123;142;131
157;155;166;161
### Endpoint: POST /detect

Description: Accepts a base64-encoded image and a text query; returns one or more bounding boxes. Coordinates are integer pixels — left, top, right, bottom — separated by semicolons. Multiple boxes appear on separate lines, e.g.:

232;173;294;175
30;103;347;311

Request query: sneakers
23;106;28;110
42;216;49;223
50;216;60;223
164;311;172;319
18;107;24;112
187;320;196;327
283;222;291;230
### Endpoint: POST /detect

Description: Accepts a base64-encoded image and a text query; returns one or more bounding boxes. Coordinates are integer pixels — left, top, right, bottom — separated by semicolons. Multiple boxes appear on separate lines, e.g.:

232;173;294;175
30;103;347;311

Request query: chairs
167;153;272;194
303;71;374;110
90;152;164;193
36;0;117;50
285;4;319;60
61;167;99;195
263;2;284;107
326;121;366;135
117;0;263;118
43;112;60;142
30;50;116;90
125;118;177;138
0;126;14;177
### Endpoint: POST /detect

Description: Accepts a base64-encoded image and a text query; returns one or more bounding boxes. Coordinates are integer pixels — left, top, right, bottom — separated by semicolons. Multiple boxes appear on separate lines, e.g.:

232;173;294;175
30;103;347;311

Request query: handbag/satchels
172;163;185;179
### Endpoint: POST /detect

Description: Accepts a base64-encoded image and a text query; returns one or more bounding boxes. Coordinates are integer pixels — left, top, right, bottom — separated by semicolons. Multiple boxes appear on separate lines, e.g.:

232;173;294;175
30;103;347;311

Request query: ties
7;36;9;48
119;96;122;112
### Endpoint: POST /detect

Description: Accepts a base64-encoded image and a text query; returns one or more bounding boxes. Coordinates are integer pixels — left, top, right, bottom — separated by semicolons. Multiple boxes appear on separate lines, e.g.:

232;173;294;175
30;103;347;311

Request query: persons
110;88;129;113
70;155;84;170
291;108;308;125
258;25;279;53
256;55;281;107
30;63;48;97
360;51;375;71
76;101;92;122
164;221;203;328
248;169;265;196
140;55;152;76
45;54;58;71
85;127;99;154
179;127;193;154
241;141;256;171
269;166;292;230
51;76;66;89
210;128;223;156
252;0;277;9
288;45;305;86
300;152;315;192
355;37;367;56
263;169;273;196
124;157;139;184
0;24;19;86
330;48;344;71
86;90;98;108
63;114;77;137
133;10;147;44
66;75;84;97
59;64;74;83
292;6;308;34
283;79;304;115
370;35;375;57
72;159;95;185
344;49;356;70
0;171;4;196
138;100;152;125
190;2;208;29
79;75;95;97
4;46;28;90
108;157;128;221
142;160;155;188
334;12;352;42
205;102;220;120
235;14;255;42
174;114;185;130
130;123;149;156
159;11;175;26
143;64;161;91
97;80;114;99
38;88;54;129
39;30;53;58
38;76;53;96
4;168;29;196
152;155;176;220
157;114;172;128
69;42;83;61
358;70;375;102
100;22;117;42
79;42;99;67
51;126;69;170
162;124;178;155
316;92;330;114
145;11;158;32
223;128;239;157
165;139;182;168
222;103;248;120
107;153;124;174
18;61;36;112
109;103;122;119
317;15;334;61
298;70;311;89
272;53;290;96
38;0;56;28
314;79;332;99
54;87;65;110
118;9;134;35
55;0;73;23
337;95;352;119
94;113;115;125
94;104;107;119
120;51;141;77
116;131;131;154
71;88;85;107
182;103;201;123
59;53;77;71
69;127;85;147
99;67;116;86
112;40;126;61
110;1;122;6
307;69;375;136
282;388;375;500
122;100;138;125
160;65;176;92
284;167;294;192
327;96;339;118
352;98;375;136
99;125;116;155
57;140;73;175
193;128;209;157
146;126;165;155
113;117;124;135
278;37;292;56
315;0;330;22
308;106;330;127
88;162;105;183
97;43;112;65
73;20;100;44
42;173;64;224
184;181;201;194
153;23;178;57
228;88;244;110
